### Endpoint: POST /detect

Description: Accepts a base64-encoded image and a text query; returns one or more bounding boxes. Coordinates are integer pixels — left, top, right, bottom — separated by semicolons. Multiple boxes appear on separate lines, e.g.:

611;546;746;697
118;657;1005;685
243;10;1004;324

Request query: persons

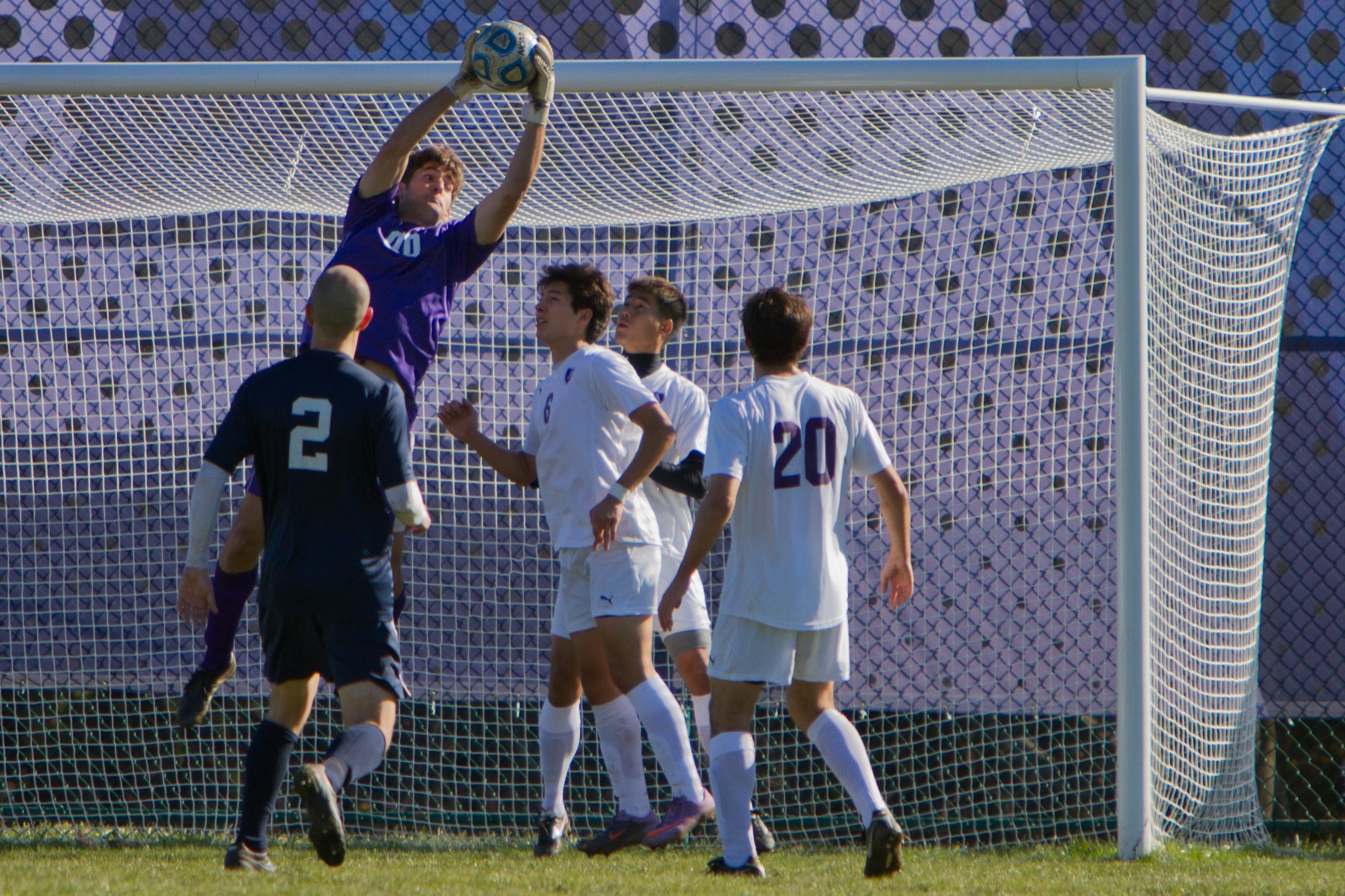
442;259;715;865
659;288;912;880
177;267;431;872
530;278;775;863
179;22;555;729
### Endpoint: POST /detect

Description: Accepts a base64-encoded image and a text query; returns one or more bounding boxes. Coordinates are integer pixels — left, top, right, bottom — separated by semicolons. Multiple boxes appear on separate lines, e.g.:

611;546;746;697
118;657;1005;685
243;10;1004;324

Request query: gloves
521;35;555;126
447;21;496;99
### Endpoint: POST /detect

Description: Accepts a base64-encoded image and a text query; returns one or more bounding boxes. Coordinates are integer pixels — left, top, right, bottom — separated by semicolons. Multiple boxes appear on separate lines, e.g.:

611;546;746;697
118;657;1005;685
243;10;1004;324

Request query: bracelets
605;478;634;509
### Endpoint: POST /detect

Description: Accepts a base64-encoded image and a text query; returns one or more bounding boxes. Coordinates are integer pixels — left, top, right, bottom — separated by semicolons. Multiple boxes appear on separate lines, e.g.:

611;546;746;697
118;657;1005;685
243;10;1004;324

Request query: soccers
471;20;540;93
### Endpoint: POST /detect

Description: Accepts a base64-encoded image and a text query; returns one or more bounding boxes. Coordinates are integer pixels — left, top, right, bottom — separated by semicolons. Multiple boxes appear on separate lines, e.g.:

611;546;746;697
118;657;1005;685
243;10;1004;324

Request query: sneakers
750;809;776;855
224;840;276;871
534;807;571;856
177;651;237;729
293;763;347;867
574;804;661;858
643;787;716;850
704;856;766;879
864;809;905;877
